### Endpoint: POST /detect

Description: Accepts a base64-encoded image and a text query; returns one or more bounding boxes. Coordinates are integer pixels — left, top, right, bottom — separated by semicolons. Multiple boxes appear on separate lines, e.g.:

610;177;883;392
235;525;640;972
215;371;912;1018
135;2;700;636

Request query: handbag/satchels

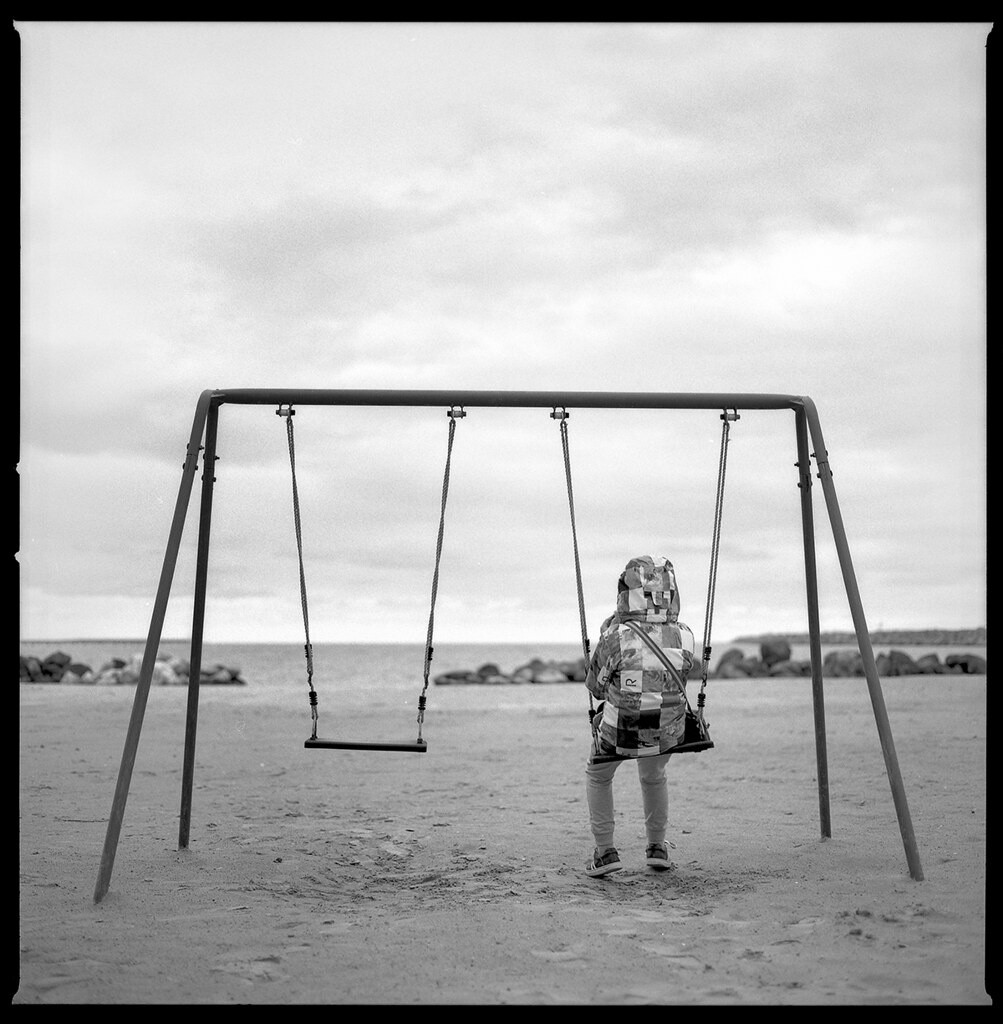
678;709;714;745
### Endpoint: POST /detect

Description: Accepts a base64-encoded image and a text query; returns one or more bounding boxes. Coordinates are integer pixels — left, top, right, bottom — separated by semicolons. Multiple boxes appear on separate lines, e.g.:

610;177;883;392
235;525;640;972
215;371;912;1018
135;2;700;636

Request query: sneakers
645;839;677;869
586;847;622;876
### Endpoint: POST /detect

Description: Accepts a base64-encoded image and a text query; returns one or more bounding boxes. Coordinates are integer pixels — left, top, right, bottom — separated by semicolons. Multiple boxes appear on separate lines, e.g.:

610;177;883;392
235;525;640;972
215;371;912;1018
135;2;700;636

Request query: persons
585;554;696;878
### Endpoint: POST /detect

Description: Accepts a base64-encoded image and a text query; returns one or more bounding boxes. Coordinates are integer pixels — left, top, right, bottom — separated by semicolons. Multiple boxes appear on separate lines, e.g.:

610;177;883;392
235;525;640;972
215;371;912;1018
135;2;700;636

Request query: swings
550;407;742;765
274;404;466;752
